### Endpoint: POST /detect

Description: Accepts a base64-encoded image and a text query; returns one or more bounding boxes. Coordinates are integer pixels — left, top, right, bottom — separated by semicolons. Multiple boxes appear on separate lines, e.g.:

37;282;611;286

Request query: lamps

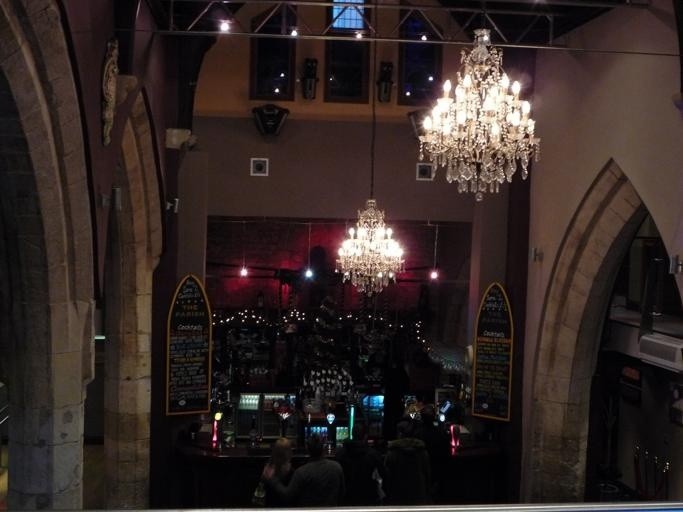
98;187;122;213
375;60;394;103
417;0;540;203
164;197;179;214
334;0;405;296
302;58;319;100
304;222;314;279
668;254;683;274
239;220;248;277
430;224;439;280
531;247;540;263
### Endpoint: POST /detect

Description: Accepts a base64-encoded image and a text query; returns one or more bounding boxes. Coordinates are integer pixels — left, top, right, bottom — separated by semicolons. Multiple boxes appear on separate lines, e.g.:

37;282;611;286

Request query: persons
260;437;346;506
334;423;382;506
378;420;432;503
261;438;293;507
413;405;449;505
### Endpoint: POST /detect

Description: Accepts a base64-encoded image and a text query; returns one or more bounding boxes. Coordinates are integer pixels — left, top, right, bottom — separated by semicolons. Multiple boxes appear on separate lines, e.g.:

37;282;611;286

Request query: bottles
249;416;259;449
223;420;236;449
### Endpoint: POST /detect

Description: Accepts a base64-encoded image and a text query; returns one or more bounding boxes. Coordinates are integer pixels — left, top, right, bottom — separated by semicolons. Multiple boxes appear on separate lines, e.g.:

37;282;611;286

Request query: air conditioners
638;334;683;373
